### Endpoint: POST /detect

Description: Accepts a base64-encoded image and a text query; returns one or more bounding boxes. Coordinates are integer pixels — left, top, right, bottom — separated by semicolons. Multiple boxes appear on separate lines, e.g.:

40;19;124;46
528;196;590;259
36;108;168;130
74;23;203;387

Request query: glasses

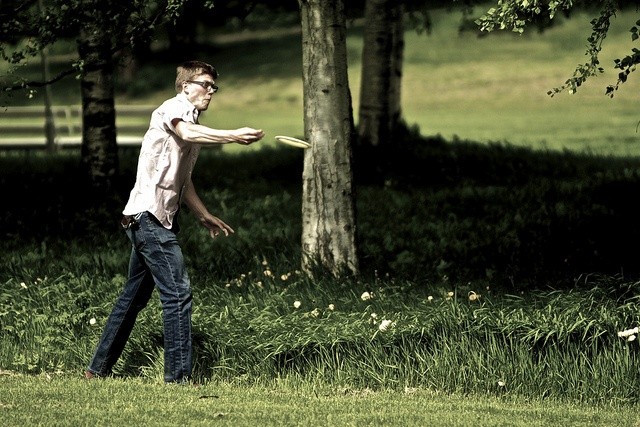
189;81;219;92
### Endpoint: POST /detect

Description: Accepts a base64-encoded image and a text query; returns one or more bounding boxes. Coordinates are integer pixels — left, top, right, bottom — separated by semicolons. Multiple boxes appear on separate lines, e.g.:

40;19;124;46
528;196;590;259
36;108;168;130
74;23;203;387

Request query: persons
84;60;266;383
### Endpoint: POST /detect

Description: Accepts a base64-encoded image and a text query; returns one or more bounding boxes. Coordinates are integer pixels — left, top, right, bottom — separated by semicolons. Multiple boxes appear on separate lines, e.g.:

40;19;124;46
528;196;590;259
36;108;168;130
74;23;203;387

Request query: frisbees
274;136;311;150
